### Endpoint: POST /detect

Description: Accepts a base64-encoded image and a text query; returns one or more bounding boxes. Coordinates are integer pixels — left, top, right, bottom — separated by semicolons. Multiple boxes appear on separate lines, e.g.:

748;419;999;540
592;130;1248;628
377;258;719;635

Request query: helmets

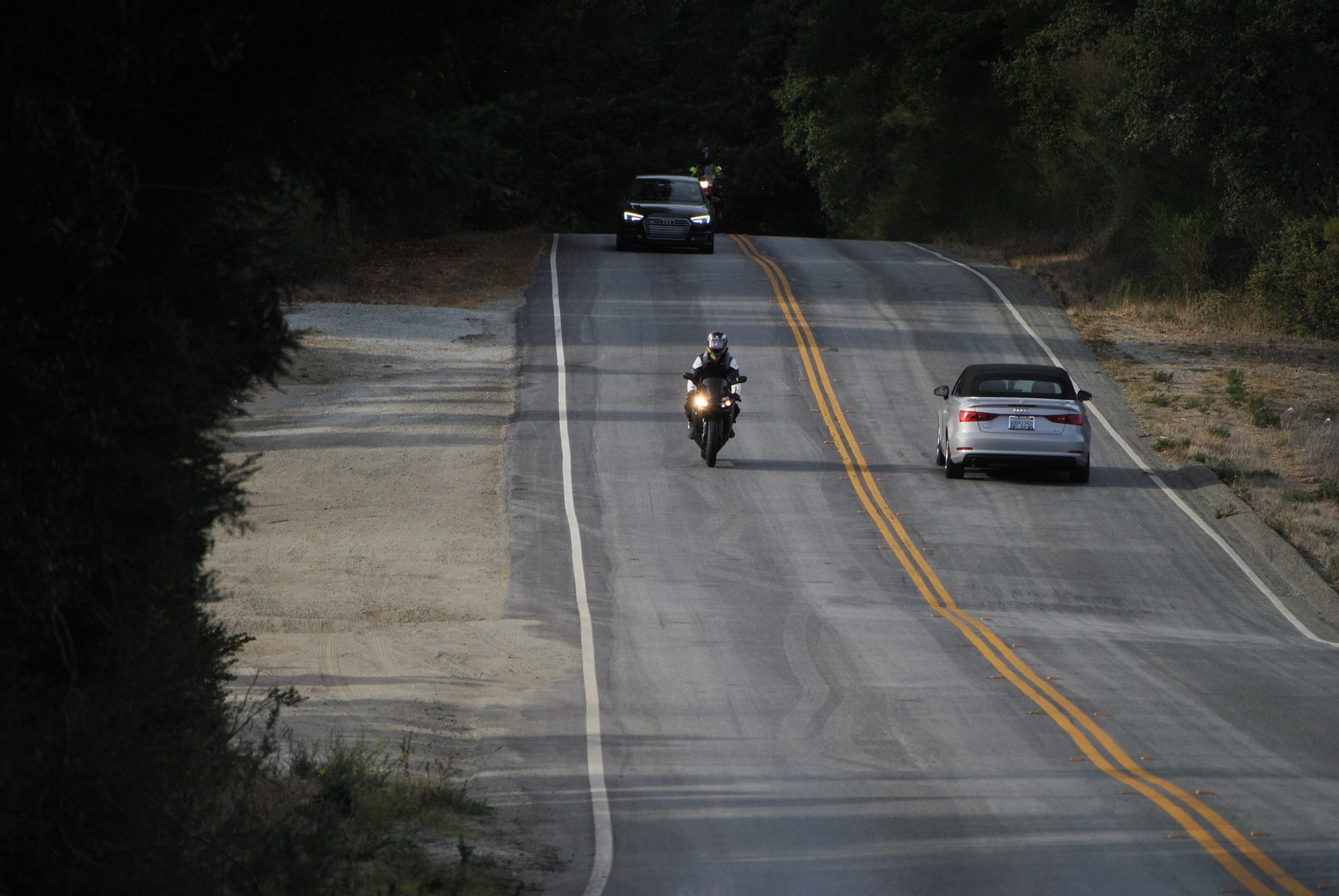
706;330;729;357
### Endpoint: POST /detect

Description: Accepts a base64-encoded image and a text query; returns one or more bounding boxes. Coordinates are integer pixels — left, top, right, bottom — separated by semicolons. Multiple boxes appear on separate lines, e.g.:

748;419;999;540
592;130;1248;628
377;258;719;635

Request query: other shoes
688;432;693;440
729;428;735;439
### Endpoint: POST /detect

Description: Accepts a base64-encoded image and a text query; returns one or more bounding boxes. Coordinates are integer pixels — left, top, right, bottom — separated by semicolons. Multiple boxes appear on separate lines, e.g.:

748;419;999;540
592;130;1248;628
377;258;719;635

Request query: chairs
979;380;1007;393
1031;381;1055;394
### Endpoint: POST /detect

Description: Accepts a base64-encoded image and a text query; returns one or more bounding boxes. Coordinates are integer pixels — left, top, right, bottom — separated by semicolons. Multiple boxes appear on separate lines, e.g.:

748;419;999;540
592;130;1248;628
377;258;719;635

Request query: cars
616;174;722;254
933;363;1093;484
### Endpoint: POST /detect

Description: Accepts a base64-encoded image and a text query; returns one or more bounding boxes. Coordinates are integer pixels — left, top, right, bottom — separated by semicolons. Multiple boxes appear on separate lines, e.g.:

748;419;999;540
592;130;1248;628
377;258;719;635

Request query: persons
685;330;743;441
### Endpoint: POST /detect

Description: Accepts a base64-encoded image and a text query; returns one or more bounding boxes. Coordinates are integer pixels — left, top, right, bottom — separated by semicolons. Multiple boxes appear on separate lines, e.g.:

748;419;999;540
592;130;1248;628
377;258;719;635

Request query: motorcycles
683;359;748;468
